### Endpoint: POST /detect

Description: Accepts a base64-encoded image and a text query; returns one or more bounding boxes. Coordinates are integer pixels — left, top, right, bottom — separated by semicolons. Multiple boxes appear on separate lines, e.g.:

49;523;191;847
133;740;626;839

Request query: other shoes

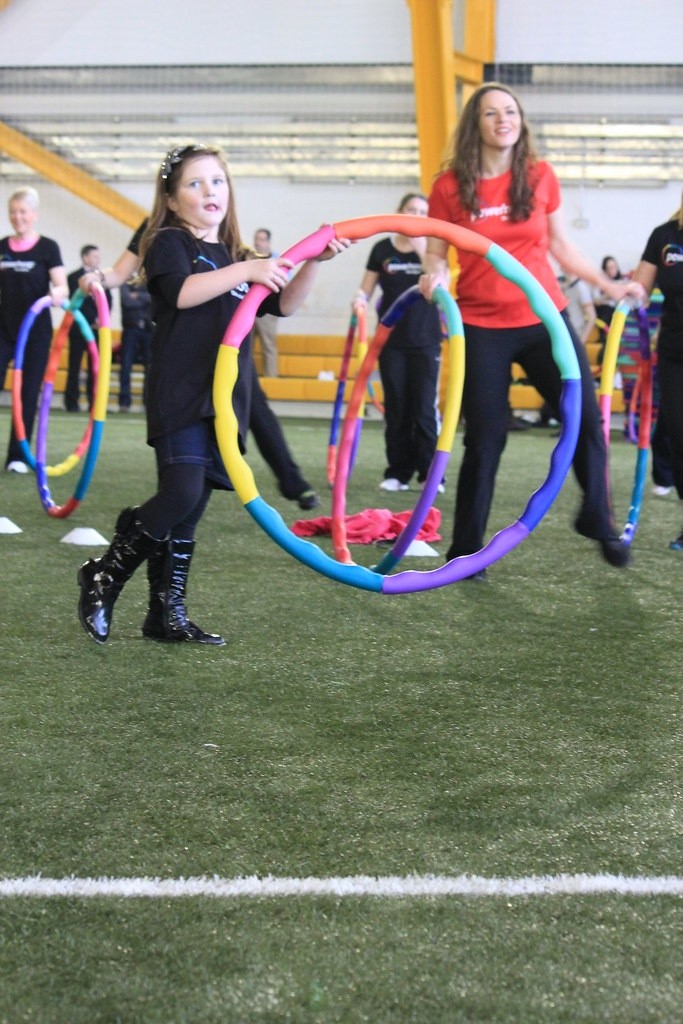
668;535;683;551
463;568;488;583
420;481;445;494
378;478;409;492
7;461;29;474
298;493;319;510
575;513;630;567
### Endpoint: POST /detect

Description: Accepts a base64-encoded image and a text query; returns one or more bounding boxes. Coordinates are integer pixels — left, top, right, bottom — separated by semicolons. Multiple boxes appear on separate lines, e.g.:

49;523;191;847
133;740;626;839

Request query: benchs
4;329;625;413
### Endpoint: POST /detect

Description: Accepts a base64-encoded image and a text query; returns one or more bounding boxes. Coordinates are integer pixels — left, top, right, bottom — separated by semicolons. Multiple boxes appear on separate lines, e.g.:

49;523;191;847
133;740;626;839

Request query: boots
77;506;171;644
142;539;227;646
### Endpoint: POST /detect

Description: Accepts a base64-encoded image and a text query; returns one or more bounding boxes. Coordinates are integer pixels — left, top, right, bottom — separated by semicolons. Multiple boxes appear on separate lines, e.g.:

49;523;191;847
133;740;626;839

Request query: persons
355;195;446;493
507;193;683;552
0;144;348;646
421;83;648;580
